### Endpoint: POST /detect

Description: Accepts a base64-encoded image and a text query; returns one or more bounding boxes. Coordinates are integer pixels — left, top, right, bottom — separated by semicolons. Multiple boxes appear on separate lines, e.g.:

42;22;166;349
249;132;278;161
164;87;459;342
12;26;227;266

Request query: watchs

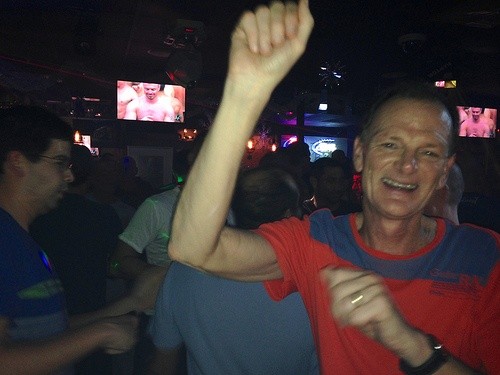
398;328;449;375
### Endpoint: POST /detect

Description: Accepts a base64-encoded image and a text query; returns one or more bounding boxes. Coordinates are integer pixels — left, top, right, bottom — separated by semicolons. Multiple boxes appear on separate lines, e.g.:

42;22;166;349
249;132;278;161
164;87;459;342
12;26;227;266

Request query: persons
422;157;464;226
168;0;500;375
123;82;166;122
0;103;363;375
456;105;496;139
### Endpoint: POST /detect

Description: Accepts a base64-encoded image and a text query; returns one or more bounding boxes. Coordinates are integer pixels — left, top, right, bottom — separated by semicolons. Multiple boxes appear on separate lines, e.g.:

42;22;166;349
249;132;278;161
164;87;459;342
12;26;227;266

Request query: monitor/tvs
456;105;499;140
115;80;188;124
